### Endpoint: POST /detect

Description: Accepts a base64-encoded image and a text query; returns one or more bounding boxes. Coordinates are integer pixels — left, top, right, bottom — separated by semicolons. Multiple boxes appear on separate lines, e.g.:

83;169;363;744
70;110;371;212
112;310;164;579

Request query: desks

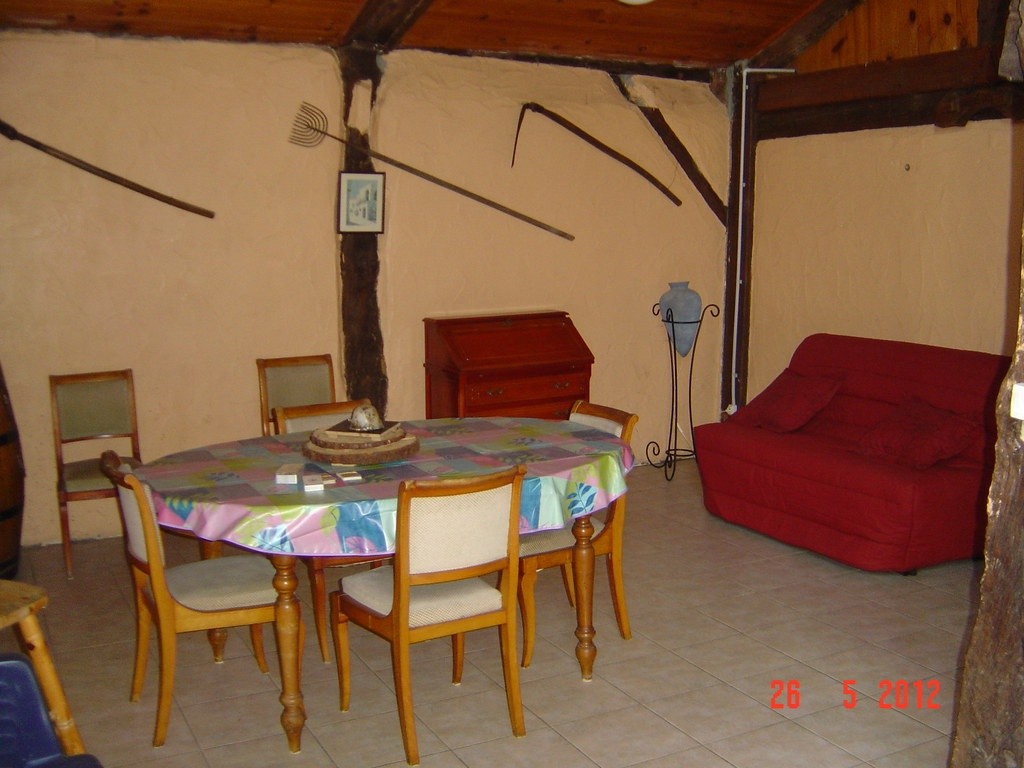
132;419;633;683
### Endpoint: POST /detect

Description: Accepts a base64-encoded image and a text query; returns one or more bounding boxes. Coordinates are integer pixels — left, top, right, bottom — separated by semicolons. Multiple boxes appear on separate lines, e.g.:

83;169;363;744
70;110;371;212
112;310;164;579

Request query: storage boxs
274;463;324;491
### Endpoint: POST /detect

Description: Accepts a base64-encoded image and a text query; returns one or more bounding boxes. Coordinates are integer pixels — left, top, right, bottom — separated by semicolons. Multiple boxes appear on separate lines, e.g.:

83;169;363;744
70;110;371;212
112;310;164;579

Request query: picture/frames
337;169;386;234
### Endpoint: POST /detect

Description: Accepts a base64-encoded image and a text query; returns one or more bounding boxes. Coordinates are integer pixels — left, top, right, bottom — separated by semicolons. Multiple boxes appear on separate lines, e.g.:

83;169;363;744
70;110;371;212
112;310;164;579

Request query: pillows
850;398;983;471
763;373;844;432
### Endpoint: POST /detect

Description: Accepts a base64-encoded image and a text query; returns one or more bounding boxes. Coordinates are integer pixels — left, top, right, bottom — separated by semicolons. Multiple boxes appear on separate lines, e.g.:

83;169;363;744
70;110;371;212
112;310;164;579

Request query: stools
0;577;84;757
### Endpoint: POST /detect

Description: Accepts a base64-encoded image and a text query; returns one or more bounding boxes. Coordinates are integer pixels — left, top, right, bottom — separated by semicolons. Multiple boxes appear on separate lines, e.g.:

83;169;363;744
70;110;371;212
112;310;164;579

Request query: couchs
691;331;1011;577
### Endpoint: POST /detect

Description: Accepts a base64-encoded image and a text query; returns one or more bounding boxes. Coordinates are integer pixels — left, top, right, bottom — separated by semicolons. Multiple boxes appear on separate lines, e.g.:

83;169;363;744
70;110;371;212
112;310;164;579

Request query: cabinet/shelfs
424;360;594;422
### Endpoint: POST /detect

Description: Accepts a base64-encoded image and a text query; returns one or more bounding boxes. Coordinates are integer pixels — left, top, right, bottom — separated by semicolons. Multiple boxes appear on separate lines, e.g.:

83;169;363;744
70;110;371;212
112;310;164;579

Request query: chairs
257;353;336;438
272;398;395;663
100;449;303;750
330;466;525;766
49;368;143;579
0;653;104;768
494;399;640;666
